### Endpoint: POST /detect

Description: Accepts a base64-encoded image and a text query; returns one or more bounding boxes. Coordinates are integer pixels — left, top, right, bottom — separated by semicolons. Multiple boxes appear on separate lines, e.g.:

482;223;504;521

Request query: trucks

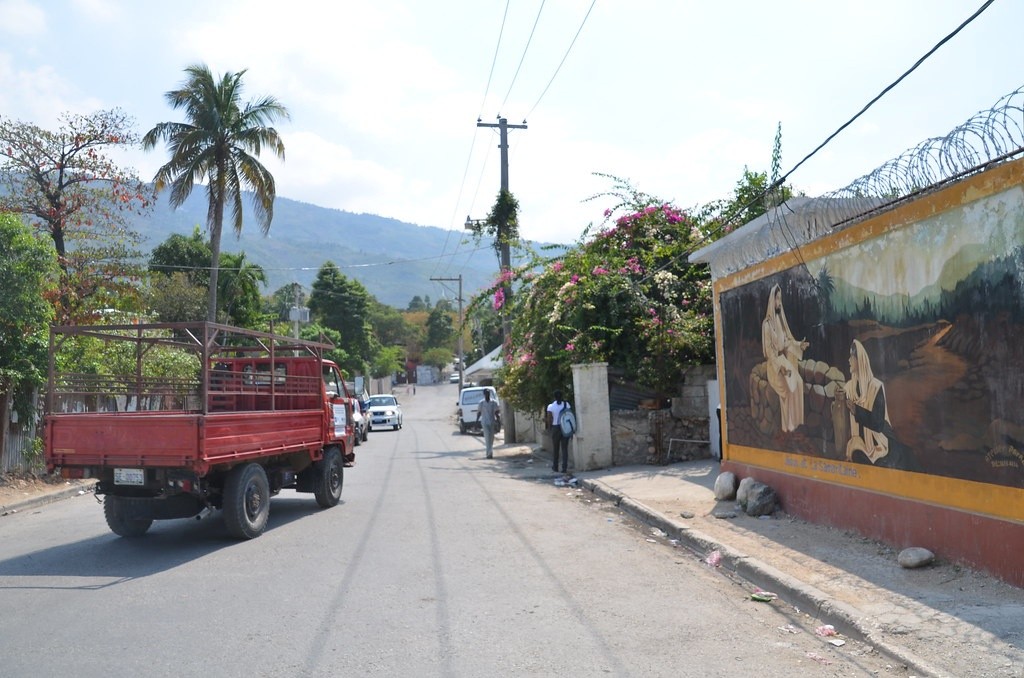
42;319;356;540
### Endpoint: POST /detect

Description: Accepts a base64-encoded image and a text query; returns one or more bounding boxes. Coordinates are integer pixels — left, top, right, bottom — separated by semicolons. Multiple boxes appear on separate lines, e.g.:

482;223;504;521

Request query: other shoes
561;470;567;474
552;466;559;472
486;455;492;460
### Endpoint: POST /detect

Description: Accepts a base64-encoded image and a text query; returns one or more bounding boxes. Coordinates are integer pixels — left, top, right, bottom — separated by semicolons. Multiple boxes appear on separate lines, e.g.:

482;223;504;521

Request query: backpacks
558;401;577;438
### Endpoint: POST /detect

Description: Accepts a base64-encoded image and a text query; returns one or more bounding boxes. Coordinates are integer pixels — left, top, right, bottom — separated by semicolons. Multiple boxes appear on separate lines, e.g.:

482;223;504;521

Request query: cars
368;394;402;431
339;397;368;446
449;374;459;383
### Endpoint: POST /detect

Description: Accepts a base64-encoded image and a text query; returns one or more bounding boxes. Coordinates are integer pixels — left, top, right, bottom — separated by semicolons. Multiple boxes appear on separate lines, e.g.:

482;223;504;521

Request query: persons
323;377;338;398
475;389;500;459
547;390;572;473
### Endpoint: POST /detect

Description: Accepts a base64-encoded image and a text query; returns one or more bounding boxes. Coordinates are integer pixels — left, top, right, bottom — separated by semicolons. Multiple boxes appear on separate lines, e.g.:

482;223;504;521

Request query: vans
456;386;500;434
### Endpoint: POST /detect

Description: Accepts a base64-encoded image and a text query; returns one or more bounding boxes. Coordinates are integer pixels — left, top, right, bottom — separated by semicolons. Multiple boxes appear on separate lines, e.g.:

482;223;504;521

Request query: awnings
464;343;504;379
